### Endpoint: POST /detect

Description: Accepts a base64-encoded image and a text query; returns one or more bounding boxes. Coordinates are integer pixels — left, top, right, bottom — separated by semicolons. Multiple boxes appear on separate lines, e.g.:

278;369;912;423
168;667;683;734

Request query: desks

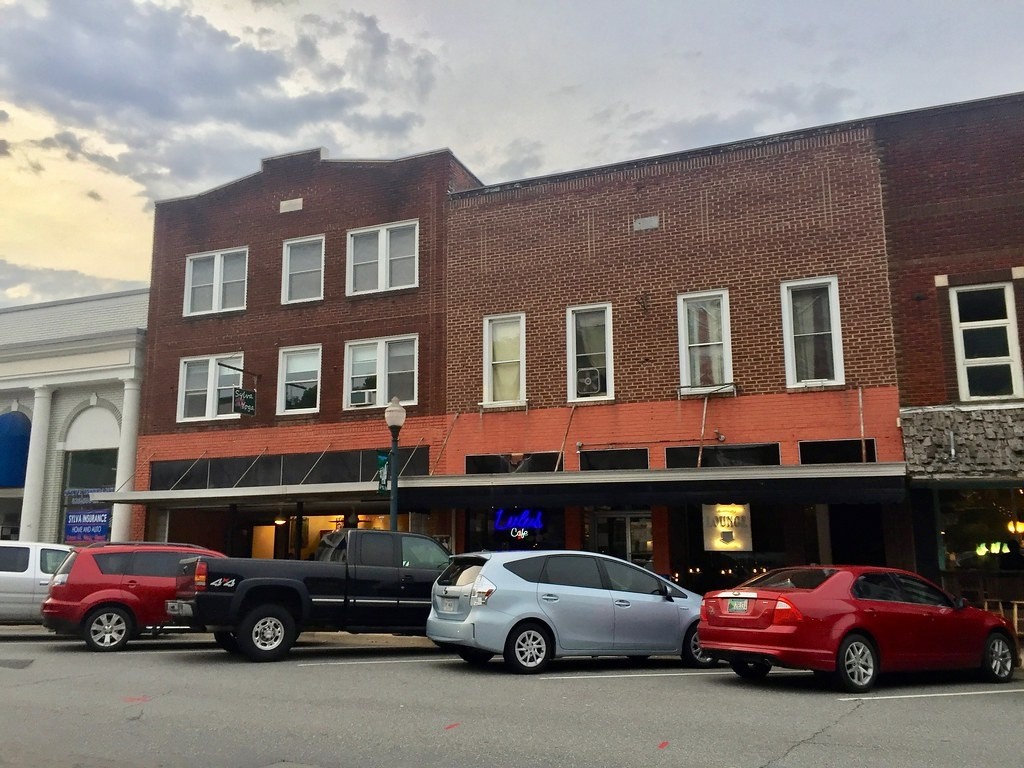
982;599;1005;617
1010;600;1024;637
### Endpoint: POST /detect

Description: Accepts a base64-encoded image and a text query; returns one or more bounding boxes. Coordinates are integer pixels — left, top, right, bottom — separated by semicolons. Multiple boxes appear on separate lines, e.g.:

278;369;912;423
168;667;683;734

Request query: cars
424;549;719;669
696;565;1020;694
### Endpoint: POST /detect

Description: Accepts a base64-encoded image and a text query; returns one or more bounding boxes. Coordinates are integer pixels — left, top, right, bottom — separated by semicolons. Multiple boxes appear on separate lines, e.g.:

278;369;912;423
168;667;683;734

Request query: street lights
384;395;406;531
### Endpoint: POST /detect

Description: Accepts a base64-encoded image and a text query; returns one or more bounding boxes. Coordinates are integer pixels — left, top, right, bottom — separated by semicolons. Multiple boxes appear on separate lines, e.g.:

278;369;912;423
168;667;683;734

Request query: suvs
40;541;231;652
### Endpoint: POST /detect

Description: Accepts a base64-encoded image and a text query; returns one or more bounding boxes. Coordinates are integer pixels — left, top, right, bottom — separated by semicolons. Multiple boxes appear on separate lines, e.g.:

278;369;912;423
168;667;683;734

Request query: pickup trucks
164;528;453;663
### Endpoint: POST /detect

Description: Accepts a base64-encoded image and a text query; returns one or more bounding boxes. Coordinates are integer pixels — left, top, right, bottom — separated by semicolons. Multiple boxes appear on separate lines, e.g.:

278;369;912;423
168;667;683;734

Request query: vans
0;540;77;626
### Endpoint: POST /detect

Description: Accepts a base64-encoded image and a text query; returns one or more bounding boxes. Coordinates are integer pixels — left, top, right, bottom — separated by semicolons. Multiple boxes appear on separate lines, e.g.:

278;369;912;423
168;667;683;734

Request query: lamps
274;507;287;526
348;507;359;524
